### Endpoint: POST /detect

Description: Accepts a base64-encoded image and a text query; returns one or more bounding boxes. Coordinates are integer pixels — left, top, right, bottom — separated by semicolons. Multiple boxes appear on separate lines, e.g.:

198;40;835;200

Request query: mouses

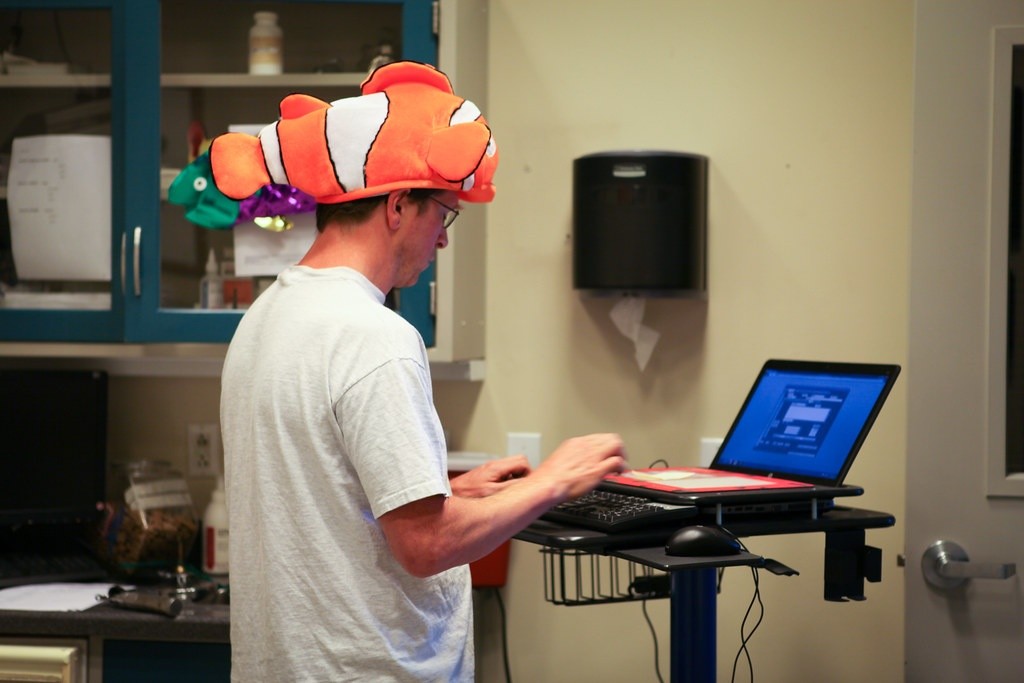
665;524;741;556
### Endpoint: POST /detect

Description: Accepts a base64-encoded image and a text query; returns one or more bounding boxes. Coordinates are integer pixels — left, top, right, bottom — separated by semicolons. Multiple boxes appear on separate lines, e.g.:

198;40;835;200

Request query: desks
0;574;230;683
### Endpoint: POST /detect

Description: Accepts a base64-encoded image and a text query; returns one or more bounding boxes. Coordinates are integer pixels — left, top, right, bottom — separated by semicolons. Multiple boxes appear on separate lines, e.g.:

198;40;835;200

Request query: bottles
247;12;284;75
199;249;225;309
202;489;230;573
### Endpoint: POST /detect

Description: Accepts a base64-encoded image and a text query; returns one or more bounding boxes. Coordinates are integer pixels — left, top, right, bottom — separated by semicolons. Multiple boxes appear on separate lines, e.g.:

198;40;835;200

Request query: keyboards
538;489;698;534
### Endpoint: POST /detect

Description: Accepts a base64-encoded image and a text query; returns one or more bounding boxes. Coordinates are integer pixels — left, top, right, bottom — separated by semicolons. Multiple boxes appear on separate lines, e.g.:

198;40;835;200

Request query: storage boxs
447;453;513;588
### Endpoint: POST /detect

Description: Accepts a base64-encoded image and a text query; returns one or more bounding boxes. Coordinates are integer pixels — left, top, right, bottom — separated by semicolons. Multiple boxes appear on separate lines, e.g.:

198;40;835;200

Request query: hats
166;61;501;228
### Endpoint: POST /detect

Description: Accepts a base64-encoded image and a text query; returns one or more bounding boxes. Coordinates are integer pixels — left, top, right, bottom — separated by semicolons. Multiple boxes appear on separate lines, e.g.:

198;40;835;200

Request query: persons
219;187;629;683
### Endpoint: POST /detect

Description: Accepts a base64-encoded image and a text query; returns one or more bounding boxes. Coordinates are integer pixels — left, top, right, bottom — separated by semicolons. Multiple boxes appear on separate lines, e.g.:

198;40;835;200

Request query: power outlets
185;424;220;478
506;434;542;470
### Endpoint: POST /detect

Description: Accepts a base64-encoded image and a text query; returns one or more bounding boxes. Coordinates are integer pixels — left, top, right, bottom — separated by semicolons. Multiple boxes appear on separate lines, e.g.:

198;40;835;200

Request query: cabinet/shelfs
0;0;491;367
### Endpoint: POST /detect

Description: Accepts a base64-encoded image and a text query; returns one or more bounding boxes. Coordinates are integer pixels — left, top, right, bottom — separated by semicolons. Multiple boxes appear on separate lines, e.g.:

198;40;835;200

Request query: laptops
592;358;902;502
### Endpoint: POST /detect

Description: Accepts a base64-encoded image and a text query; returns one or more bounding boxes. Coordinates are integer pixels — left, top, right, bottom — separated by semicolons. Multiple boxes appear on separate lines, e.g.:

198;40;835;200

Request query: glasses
415;188;461;229
